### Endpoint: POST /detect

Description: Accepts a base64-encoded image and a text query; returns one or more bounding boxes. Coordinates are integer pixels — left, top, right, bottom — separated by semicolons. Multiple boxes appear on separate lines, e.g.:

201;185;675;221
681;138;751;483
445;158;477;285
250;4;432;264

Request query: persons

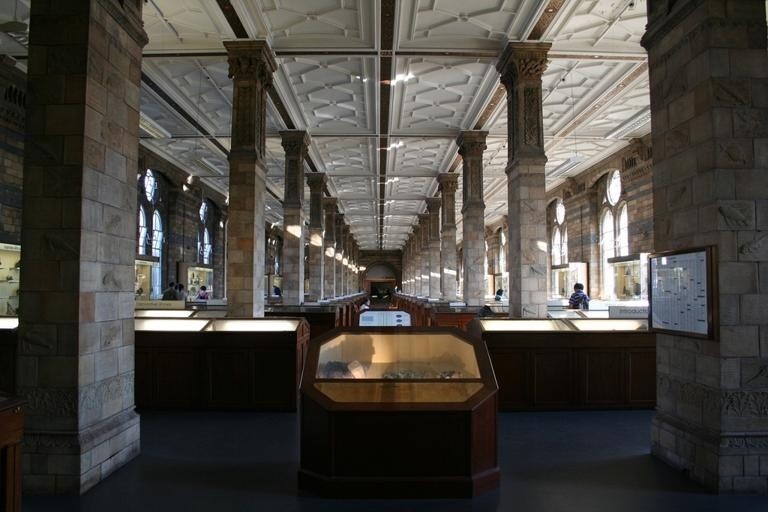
194;286;210;299
272;284;281;296
569;283;590;309
494;289;503;301
160;282;178;300
174;283;186;300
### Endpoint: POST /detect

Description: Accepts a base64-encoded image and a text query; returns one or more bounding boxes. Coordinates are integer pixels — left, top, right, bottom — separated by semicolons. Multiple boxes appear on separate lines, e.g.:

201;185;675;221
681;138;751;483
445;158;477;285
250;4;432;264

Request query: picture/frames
646;244;720;342
